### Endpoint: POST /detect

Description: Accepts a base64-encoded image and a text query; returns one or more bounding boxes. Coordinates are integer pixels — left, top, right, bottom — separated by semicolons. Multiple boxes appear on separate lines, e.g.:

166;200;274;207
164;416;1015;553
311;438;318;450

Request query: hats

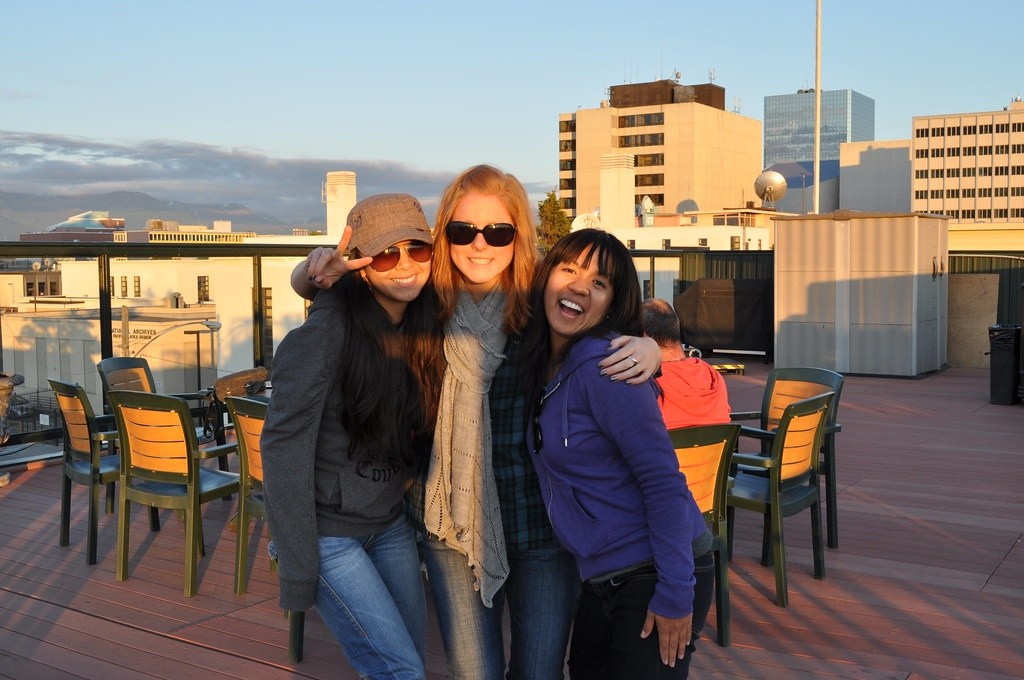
346;193;436;256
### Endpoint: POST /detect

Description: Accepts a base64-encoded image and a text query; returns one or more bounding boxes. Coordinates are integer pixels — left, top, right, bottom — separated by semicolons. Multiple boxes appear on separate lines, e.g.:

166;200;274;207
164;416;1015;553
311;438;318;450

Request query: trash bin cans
987;323;1023;407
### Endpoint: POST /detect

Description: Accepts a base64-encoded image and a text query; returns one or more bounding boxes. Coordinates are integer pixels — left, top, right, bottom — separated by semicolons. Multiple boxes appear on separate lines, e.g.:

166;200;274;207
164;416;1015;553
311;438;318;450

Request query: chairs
222;395;292;620
727;365;845;566
106;390;251;600
665;423;744;648
726;390;836;609
94;356;234;501
44;377;208;566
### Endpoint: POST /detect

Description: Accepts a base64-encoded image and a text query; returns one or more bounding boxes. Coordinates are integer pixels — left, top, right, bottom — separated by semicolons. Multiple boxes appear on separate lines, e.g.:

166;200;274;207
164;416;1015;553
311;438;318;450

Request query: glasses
358;239;432;272
445;221;514;246
531;387;546;451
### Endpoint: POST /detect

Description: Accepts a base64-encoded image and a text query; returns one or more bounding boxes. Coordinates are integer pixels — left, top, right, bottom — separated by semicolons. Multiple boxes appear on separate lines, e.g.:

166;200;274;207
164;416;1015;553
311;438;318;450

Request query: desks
212;379;273;413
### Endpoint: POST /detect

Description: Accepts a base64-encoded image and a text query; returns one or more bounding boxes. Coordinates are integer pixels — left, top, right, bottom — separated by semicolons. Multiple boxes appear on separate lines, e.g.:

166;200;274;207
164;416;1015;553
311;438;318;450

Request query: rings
628;355;638;366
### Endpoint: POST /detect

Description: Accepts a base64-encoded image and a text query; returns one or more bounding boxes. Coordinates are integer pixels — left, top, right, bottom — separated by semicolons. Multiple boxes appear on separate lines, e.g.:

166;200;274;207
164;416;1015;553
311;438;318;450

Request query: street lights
124;319;224;358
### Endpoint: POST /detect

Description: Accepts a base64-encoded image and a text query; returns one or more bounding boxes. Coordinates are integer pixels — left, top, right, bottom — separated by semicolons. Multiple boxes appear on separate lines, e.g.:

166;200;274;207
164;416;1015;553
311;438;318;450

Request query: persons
258;165;435;680
528;227;713;680
636;296;729;433
295;165;661;680
